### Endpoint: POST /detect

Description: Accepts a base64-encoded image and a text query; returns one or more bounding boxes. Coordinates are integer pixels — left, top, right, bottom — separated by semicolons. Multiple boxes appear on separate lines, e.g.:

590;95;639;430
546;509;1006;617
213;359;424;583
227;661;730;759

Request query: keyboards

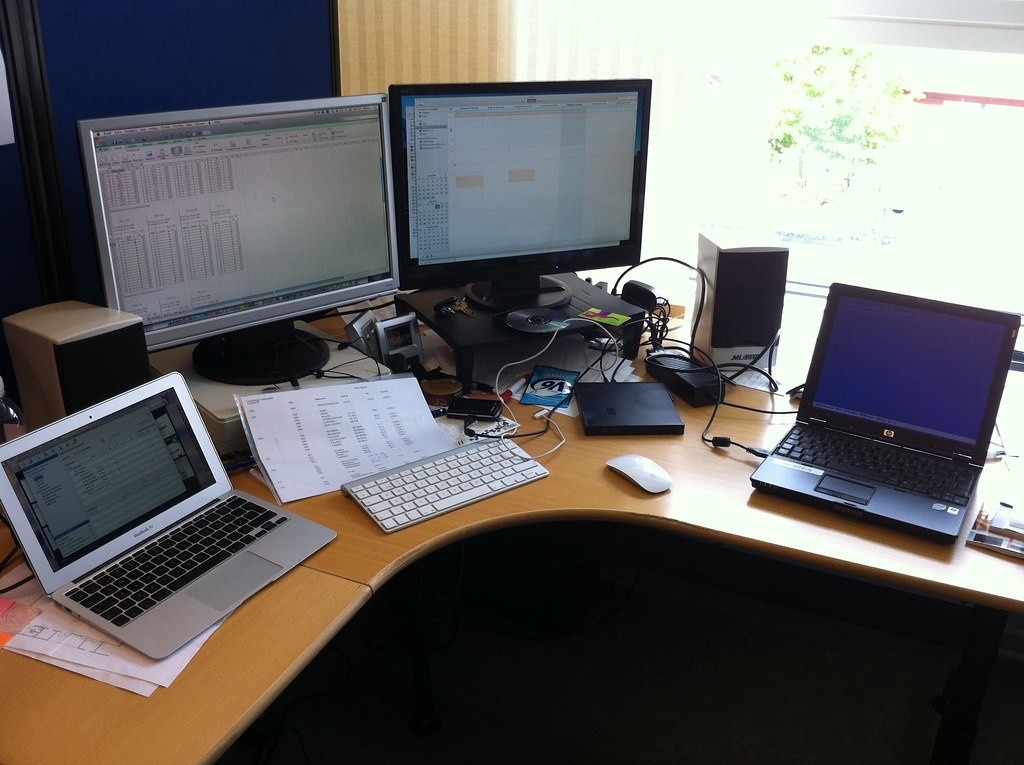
340;433;550;534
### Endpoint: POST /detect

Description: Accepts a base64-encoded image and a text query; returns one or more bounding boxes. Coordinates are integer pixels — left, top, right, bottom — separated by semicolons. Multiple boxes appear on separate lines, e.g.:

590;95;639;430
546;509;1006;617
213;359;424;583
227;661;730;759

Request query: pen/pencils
500;374;529;402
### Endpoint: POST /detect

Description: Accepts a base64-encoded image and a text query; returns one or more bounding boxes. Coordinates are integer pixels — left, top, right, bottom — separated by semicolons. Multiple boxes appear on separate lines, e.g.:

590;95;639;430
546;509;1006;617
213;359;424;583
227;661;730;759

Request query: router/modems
572;381;685;436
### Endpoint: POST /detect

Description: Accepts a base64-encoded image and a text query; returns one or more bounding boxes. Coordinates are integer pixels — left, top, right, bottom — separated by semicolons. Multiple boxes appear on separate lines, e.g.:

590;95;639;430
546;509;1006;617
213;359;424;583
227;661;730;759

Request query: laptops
0;369;341;662
749;283;1021;547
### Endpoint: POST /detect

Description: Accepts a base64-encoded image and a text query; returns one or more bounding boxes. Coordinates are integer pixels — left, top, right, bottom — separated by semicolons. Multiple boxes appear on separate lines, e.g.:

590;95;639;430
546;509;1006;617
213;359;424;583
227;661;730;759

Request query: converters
644;346;725;408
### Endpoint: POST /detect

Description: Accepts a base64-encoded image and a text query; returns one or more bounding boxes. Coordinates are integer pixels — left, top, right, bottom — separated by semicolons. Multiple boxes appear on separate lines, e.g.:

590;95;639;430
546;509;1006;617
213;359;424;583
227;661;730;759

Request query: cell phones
447;397;504;422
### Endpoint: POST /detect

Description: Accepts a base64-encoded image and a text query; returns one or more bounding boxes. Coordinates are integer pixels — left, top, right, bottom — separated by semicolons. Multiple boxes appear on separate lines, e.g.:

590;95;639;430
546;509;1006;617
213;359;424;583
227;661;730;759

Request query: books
968;480;1024;560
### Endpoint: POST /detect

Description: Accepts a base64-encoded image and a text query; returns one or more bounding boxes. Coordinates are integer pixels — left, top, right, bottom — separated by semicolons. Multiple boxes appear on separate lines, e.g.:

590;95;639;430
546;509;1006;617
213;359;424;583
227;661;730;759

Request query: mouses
605;454;673;494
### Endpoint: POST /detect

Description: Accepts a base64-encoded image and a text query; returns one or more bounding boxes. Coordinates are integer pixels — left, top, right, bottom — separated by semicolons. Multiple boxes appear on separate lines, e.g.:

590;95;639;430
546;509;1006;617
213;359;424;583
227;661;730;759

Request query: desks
0;280;1023;760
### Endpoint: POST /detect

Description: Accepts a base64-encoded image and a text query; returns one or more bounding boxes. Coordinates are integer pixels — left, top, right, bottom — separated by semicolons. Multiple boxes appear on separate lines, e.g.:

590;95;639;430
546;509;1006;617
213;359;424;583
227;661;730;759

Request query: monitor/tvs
77;77;651;353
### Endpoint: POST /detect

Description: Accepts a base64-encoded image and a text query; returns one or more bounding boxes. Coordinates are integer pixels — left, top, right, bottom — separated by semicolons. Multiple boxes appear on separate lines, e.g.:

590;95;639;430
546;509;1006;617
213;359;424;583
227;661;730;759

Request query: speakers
691;227;790;372
3;299;154;434
